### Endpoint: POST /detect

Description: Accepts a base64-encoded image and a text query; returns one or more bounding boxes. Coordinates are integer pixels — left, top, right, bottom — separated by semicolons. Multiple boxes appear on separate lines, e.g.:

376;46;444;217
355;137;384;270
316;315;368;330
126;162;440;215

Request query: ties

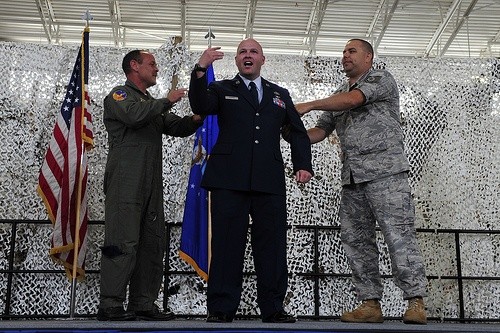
249;82;260;105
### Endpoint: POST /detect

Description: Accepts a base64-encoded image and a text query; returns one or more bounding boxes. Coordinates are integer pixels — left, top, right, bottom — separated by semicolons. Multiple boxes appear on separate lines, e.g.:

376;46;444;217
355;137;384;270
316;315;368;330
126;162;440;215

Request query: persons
96;50;206;322
189;38;314;322
294;39;430;324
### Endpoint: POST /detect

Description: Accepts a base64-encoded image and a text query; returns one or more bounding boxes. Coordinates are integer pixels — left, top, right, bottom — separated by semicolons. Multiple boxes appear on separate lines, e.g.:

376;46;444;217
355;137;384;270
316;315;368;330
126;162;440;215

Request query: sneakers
340;300;384;323
402;299;427;325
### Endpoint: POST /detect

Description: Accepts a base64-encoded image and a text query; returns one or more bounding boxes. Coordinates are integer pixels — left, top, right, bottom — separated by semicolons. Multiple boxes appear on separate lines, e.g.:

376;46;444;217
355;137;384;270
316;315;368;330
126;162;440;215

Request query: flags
180;64;220;283
37;28;93;283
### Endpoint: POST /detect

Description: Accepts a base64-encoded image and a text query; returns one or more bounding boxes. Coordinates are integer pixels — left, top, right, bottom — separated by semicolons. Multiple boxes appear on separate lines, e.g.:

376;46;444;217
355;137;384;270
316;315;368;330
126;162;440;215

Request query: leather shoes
261;312;296;323
127;307;175;321
205;315;228;323
97;307;135;321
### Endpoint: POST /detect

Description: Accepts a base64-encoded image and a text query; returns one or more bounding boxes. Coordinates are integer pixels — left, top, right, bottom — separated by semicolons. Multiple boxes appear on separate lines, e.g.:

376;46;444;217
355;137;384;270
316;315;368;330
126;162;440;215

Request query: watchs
194;63;207;72
